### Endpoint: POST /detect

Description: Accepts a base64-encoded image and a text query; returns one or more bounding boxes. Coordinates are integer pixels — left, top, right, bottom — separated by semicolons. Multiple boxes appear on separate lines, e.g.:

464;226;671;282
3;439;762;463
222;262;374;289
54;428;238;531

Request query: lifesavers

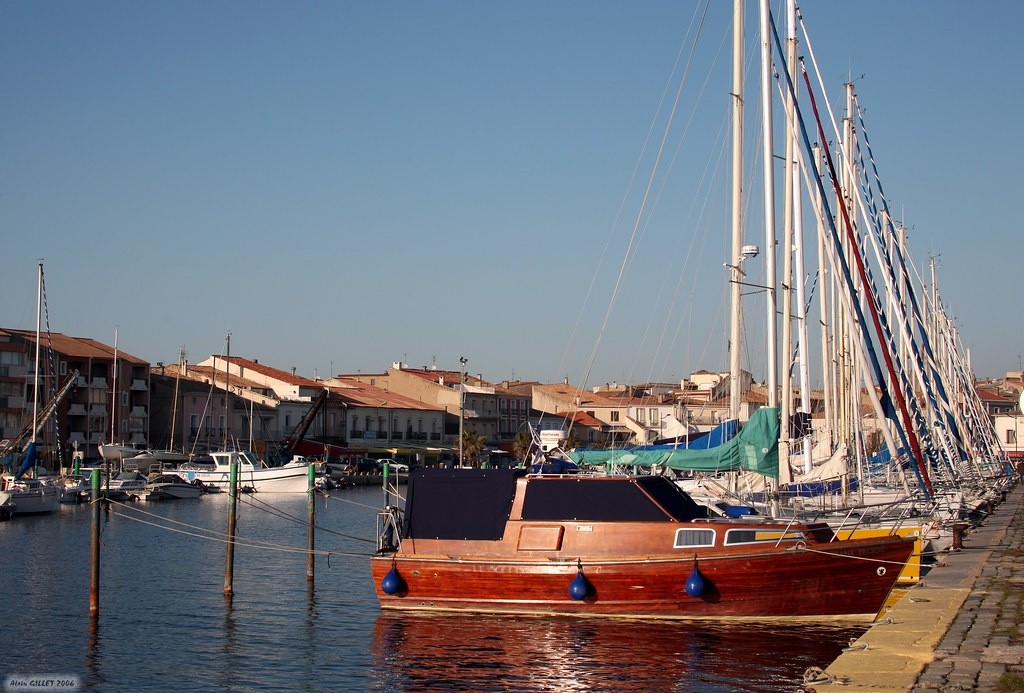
343;465;353;474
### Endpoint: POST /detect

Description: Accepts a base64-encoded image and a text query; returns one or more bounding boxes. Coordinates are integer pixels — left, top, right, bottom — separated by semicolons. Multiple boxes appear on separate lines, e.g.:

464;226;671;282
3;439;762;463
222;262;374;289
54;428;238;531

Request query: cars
376;458;411;473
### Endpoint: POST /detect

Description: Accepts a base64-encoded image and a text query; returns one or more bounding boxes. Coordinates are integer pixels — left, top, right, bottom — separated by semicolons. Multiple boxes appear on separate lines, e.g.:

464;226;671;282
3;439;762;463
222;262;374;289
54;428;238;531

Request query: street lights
459;355;469;470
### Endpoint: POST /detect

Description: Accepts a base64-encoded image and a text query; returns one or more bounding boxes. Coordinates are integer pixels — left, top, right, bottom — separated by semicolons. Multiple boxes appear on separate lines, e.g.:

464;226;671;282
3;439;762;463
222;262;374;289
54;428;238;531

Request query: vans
339;456;384;476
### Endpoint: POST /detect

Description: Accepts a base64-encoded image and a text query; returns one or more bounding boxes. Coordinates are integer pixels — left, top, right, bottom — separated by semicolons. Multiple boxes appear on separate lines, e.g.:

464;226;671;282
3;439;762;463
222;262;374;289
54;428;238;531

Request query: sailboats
375;1;1018;634
0;256;333;521
